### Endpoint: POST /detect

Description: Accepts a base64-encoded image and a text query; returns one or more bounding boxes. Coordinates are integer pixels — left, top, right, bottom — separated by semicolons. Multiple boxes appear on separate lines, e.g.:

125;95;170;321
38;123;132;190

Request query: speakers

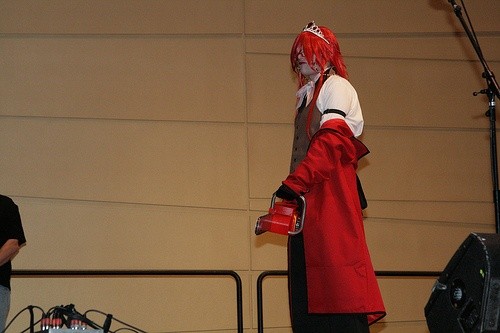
423;232;500;333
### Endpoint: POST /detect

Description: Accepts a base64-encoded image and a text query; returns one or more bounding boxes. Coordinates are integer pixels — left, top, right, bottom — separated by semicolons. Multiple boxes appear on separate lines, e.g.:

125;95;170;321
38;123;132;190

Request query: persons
0;194;27;333
275;25;387;333
